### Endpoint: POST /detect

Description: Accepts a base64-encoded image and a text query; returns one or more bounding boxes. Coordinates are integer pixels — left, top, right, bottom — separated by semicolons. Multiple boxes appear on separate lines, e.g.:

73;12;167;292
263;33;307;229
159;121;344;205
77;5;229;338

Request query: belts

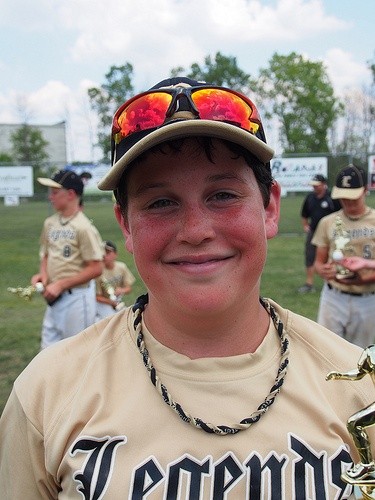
327;284;375;297
68;289;72;295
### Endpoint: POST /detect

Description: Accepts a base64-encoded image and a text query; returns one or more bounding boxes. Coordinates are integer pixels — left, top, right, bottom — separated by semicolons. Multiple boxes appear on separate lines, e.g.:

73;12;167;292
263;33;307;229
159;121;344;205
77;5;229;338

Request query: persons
31;169;104;350
340;257;375;270
0;78;375;500
300;173;341;292
95;242;136;323
308;163;375;350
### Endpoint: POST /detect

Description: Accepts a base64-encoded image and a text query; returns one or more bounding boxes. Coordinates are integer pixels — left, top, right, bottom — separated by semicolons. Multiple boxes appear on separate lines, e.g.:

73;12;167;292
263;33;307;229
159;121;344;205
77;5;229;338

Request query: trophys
333;216;354;281
8;281;61;305
325;343;375;500
100;278;125;310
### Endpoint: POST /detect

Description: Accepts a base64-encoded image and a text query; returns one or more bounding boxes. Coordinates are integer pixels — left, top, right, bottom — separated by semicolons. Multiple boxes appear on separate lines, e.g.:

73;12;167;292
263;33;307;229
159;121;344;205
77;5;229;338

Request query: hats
36;169;84;194
309;175;324;186
102;240;117;253
99;77;275;191
330;164;365;200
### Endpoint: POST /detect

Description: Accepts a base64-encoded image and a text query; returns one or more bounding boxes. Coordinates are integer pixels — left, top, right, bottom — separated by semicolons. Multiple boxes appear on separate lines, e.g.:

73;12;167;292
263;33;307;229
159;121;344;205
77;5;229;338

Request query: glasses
110;85;267;165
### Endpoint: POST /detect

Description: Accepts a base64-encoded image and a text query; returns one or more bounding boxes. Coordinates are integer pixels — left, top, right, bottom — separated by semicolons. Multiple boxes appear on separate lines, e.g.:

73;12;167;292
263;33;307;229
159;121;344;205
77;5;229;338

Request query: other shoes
296;284;316;294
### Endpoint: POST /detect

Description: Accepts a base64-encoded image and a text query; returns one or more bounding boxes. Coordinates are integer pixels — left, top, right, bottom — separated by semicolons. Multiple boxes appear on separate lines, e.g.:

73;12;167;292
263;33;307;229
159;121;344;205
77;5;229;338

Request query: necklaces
343;208;372;221
58;210;80;225
132;293;292;434
